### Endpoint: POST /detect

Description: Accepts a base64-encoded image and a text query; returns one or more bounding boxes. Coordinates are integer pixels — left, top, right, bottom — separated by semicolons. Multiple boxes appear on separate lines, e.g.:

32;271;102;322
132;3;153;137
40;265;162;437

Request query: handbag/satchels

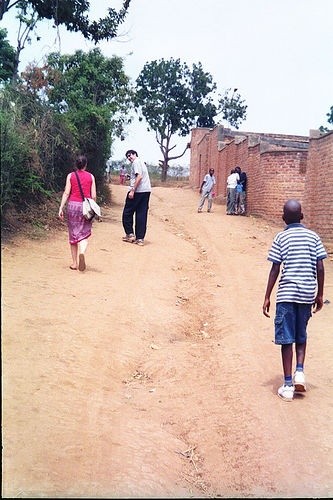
83;197;101;223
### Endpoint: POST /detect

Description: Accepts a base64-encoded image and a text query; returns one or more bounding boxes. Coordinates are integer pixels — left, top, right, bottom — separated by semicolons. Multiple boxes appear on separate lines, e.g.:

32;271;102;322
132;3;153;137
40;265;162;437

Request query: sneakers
122;234;136;243
293;371;306;392
133;239;144;246
277;384;295;402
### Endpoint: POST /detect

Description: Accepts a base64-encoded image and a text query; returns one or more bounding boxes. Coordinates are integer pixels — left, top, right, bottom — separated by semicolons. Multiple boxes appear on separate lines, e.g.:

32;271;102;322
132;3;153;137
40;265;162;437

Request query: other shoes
79;254;86;271
70;264;77;270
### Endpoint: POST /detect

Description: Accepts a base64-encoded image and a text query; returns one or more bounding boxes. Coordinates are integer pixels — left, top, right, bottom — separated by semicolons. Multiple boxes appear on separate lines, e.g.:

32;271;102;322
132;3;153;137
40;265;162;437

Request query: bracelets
131;187;136;191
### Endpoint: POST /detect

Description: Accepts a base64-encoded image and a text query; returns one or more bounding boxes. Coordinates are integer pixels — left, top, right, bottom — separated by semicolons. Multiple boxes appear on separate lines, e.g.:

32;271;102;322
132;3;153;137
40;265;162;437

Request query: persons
226;167;247;215
58;155;96;271
262;200;327;402
197;168;217;212
119;150;151;245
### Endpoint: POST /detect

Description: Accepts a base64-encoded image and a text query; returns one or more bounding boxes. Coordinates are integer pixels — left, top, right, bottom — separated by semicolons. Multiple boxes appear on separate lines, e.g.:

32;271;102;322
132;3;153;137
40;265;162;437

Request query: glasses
127;155;130;158
